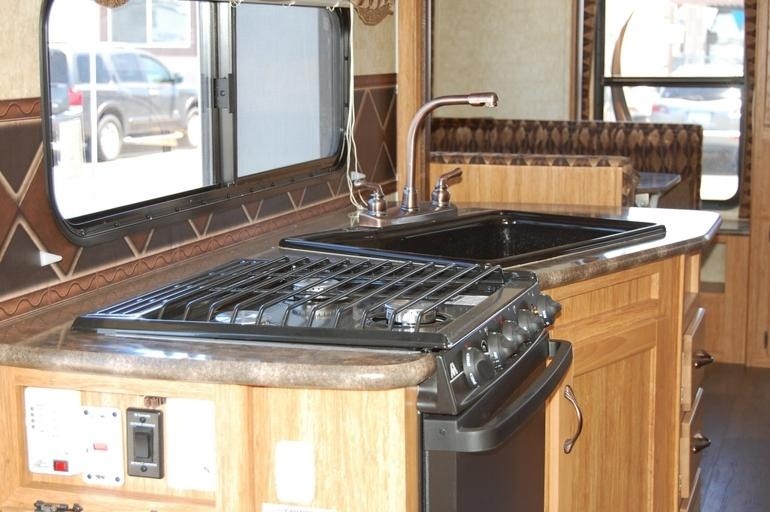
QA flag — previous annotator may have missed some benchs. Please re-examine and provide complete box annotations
[427,152,641,208]
[432,117,704,207]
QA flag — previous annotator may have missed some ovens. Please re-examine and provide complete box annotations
[421,332,575,512]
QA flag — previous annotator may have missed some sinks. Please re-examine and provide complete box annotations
[278,208,667,271]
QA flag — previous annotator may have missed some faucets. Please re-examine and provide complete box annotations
[400,92,498,215]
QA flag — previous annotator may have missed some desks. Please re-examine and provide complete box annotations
[635,172,682,207]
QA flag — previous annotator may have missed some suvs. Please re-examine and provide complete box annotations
[47,39,198,161]
[647,62,742,169]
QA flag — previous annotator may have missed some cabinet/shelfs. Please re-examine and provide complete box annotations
[545,251,713,512]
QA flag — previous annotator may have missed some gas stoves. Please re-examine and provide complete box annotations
[71,256,562,417]
